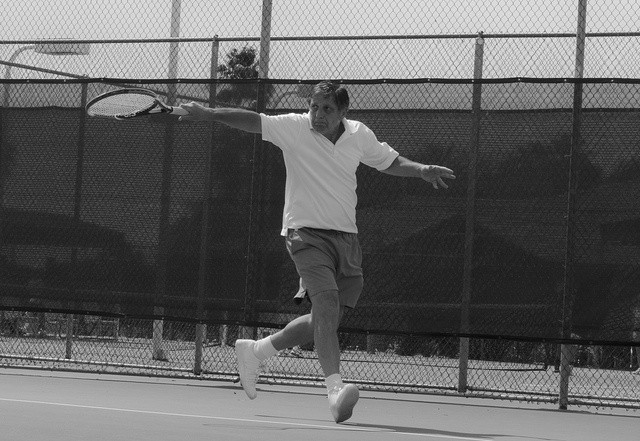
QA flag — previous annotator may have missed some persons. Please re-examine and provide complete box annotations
[176,78,457,425]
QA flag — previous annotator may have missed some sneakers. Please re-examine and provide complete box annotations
[235,338,264,400]
[328,382,360,424]
